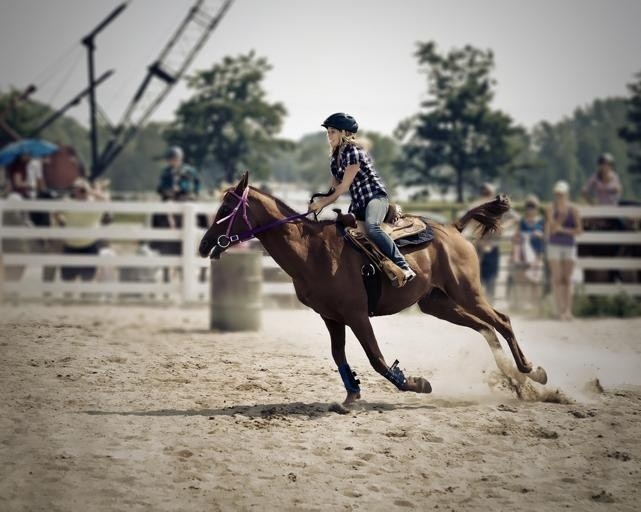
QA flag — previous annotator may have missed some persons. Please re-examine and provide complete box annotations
[545,179,583,323]
[5,147,112,302]
[513,193,549,323]
[308,111,419,287]
[580,150,640,286]
[469,182,521,310]
[117,146,210,296]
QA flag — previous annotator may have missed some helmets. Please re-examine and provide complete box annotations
[166,147,184,160]
[320,113,358,134]
[597,152,613,165]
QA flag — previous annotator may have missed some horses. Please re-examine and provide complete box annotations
[197,169,548,406]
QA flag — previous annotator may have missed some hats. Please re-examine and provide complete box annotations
[553,180,566,193]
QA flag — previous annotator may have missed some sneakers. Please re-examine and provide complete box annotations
[391,267,417,287]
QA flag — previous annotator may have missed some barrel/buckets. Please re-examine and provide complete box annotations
[211,251,263,331]
